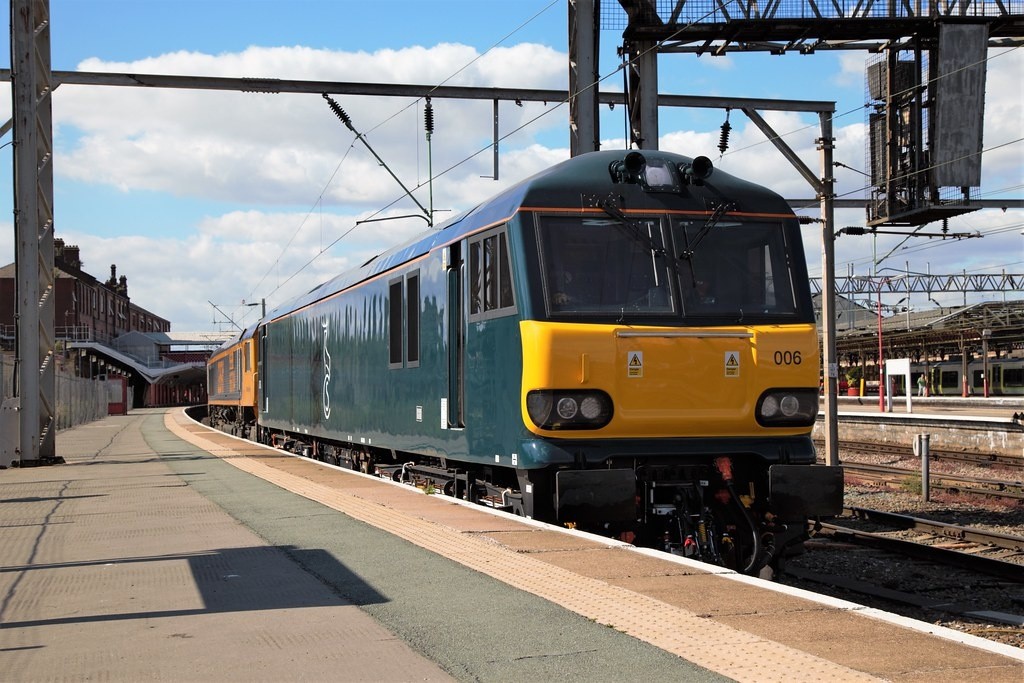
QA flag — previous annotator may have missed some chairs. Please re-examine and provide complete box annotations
[560,255,624,305]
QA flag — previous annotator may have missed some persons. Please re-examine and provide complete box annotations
[917,374,926,397]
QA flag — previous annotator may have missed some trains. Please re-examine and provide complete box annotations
[893,356,1024,397]
[208,149,846,580]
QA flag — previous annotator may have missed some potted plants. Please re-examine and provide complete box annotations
[844,365,866,396]
